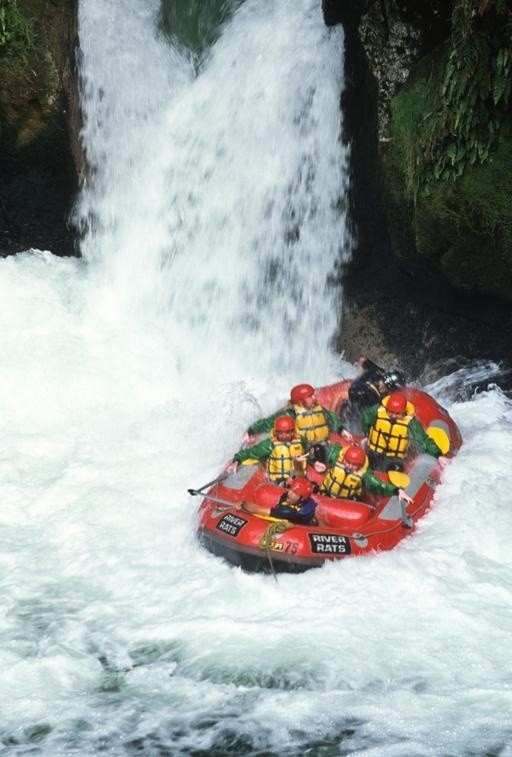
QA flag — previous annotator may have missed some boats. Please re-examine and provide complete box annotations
[197,377,462,577]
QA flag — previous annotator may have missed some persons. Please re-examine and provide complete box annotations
[359,391,451,474]
[295,441,415,504]
[239,383,358,449]
[343,355,405,415]
[232,479,318,526]
[227,414,326,487]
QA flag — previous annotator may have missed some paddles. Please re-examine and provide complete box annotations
[188,458,260,497]
[426,428,450,456]
[380,395,415,418]
[387,469,411,530]
[186,488,291,525]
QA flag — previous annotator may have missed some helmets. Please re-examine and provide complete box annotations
[290,384,314,404]
[274,416,295,431]
[386,393,406,413]
[383,371,404,392]
[345,446,366,465]
[290,478,312,501]
